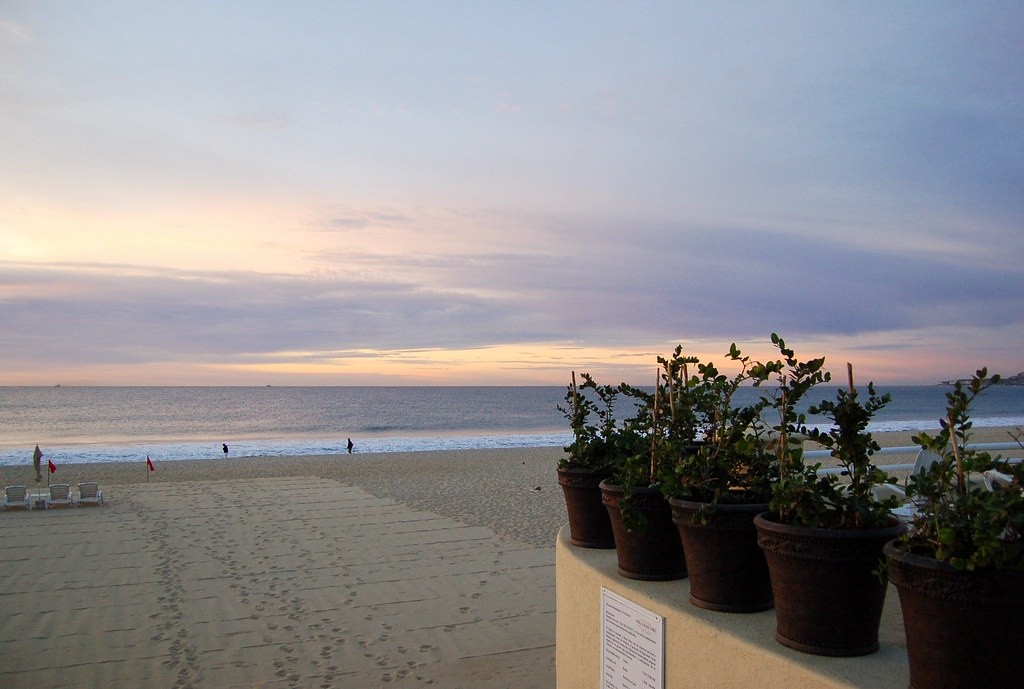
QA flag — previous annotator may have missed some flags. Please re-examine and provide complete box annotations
[147,458,155,471]
[49,461,56,473]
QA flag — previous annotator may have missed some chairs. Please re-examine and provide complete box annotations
[46,484,73,510]
[876,442,953,503]
[76,482,104,508]
[3,486,29,511]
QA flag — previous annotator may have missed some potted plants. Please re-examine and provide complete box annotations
[554,369,647,549]
[650,343,784,613]
[881,367,1024,689]
[752,333,909,658]
[598,345,699,581]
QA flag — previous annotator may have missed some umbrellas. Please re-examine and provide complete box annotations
[33,445,43,481]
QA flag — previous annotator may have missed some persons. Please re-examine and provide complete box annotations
[347,438,353,453]
[223,444,228,459]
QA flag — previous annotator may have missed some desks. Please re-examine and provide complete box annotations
[30,494,48,510]
